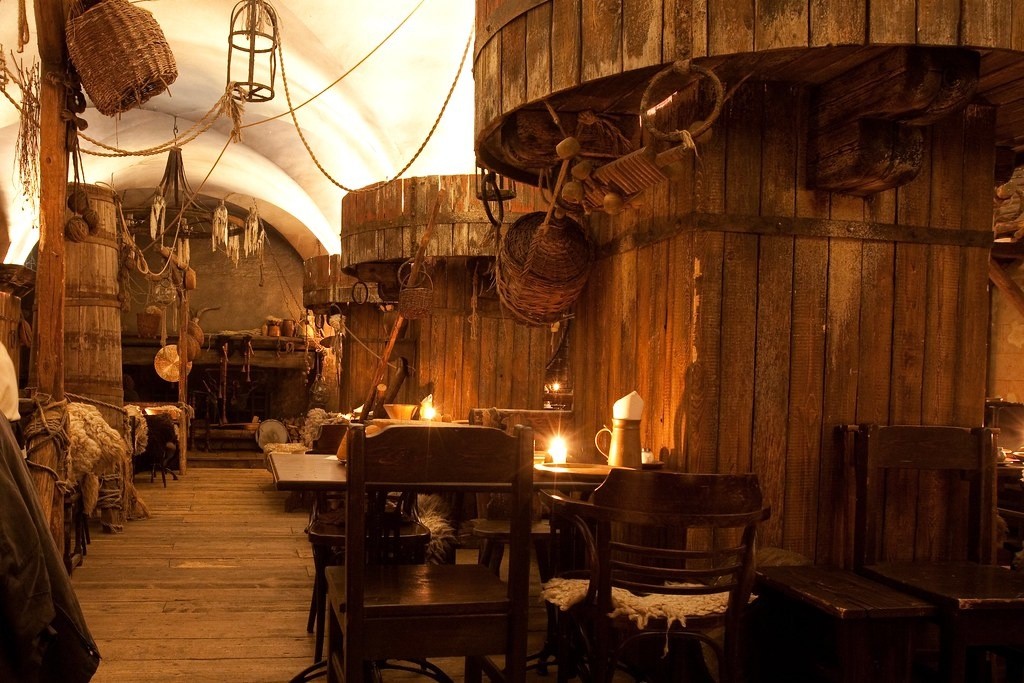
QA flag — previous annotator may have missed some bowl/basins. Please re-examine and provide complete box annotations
[383,404,419,420]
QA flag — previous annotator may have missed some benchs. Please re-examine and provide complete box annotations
[753,564,935,683]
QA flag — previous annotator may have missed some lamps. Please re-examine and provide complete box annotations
[542,353,576,411]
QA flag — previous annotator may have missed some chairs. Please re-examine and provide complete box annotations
[856,422,1024,683]
[324,424,536,683]
[537,466,771,683]
[469,406,576,570]
[307,425,431,664]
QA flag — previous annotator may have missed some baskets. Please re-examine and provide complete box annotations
[65,2,179,115]
[399,272,434,318]
[499,212,596,322]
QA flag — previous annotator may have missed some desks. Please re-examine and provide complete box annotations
[268,452,603,487]
[997,457,1024,547]
[120,333,319,425]
[123,402,190,476]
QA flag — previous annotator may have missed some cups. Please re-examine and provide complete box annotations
[595,419,642,470]
